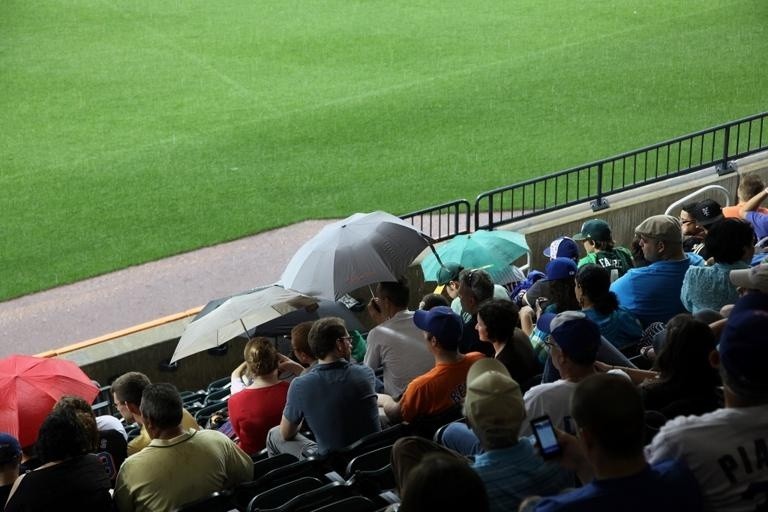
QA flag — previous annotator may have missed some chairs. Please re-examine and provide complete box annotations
[89,343,657,512]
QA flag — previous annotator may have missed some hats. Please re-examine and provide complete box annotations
[464,355,528,434]
[694,198,723,224]
[719,294,767,385]
[633,213,683,242]
[414,305,465,351]
[541,218,613,284]
[536,310,602,366]
[436,262,464,286]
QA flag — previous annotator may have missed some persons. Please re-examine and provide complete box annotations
[1,372,254,511]
[228,175,768,511]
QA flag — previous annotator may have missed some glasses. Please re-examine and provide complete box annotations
[337,334,354,345]
[677,216,692,226]
[543,335,557,349]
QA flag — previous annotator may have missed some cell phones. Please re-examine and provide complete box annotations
[529,415,561,461]
